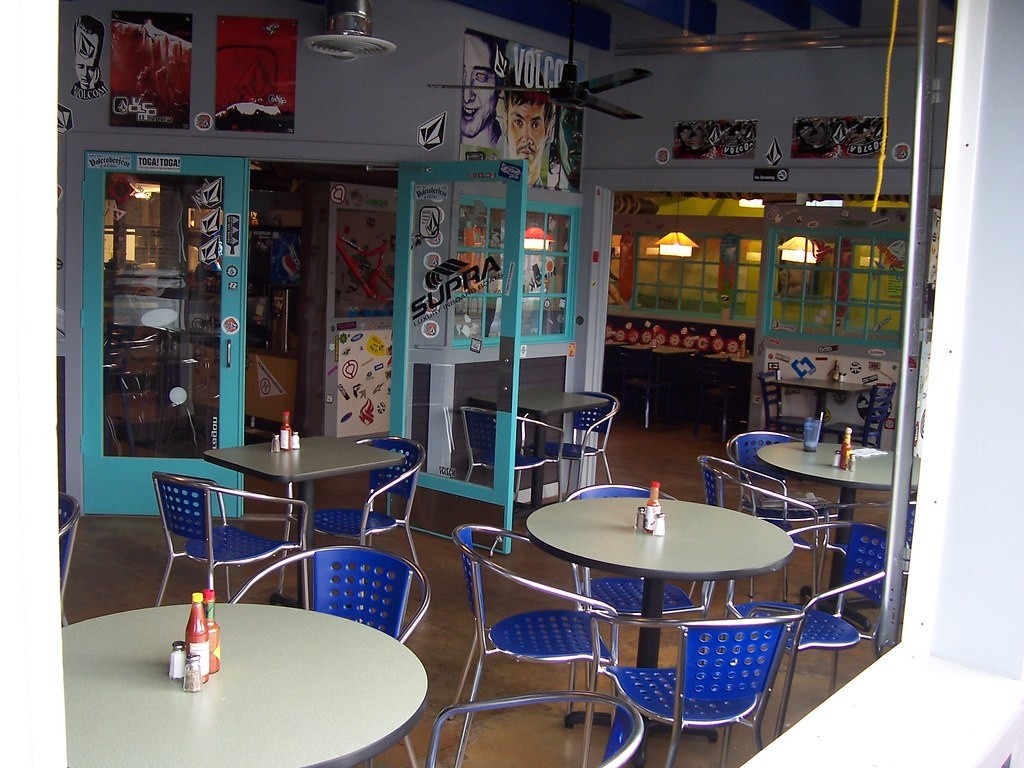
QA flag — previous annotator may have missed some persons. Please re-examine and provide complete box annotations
[462,28,583,193]
[106,242,136,271]
[189,264,217,301]
[248,234,267,296]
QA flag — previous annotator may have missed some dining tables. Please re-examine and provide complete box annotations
[63,604,428,768]
[527,499,795,768]
[200,436,405,610]
[467,389,609,519]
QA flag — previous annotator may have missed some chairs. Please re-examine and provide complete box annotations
[60,372,920,768]
[619,351,739,444]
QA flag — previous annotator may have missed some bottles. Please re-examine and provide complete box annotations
[291,432,300,449]
[833,427,856,472]
[832,361,845,383]
[653,513,666,537]
[183,654,202,692]
[202,589,220,674]
[185,593,209,684]
[271,434,280,453]
[635,507,644,531]
[169,640,186,680]
[644,481,662,533]
[776,369,783,381]
[280,411,292,451]
[606,332,746,358]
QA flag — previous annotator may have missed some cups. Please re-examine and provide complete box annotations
[803,417,822,452]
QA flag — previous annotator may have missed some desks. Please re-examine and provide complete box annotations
[756,443,921,632]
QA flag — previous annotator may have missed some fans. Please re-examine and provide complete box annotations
[426,0,653,121]
